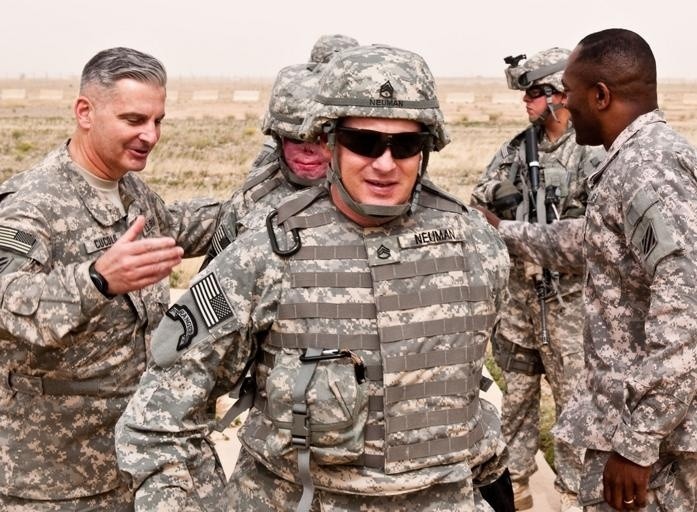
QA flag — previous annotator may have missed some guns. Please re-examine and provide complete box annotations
[487,127,560,346]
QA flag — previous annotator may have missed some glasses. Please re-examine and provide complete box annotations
[336,125,426,160]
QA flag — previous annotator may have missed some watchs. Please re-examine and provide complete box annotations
[89,264,117,299]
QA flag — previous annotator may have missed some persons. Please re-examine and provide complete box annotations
[113,44,516,512]
[476,27,697,512]
[0,47,225,512]
[253,34,359,167]
[470,46,603,512]
[166,61,326,258]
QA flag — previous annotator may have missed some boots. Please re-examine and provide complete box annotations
[510,479,532,509]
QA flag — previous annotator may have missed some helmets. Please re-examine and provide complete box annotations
[306,35,359,61]
[297,42,450,152]
[262,61,324,142]
[526,46,572,95]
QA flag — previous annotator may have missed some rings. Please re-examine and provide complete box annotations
[623,496,637,504]
